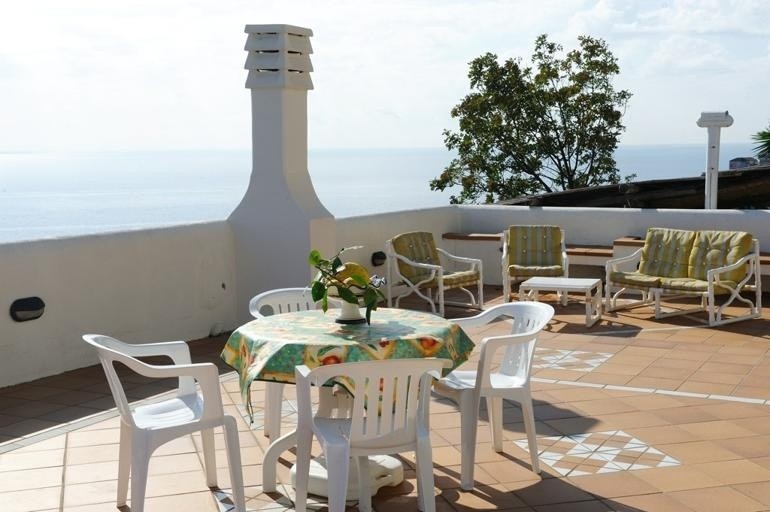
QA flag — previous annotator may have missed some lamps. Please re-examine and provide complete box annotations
[10,296,46,322]
[372,251,386,267]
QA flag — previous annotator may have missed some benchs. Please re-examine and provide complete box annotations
[566,246,613,267]
[614,235,645,272]
[443,230,505,286]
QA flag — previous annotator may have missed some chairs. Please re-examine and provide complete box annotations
[605,229,762,328]
[412,301,556,491]
[385,230,484,318]
[248,287,347,447]
[82,333,246,511]
[502,224,569,307]
[263,357,453,512]
